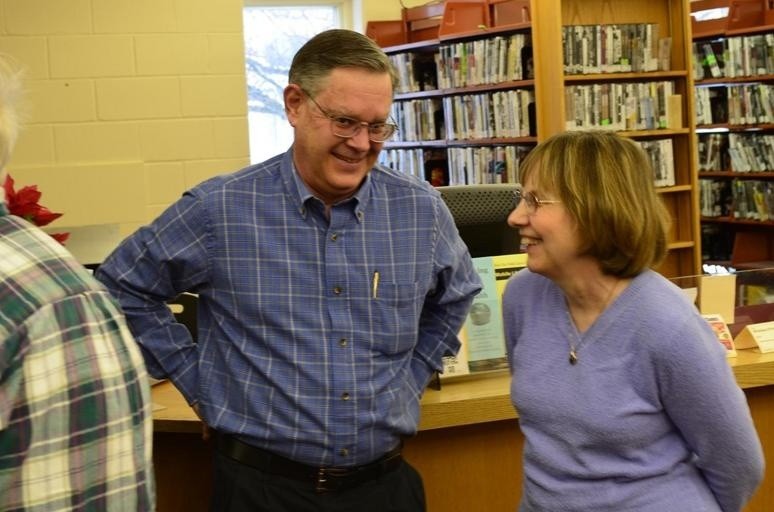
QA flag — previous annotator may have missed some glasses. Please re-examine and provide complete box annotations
[300,89,399,143]
[511,191,565,211]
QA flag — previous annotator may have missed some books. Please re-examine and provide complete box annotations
[561,23,683,189]
[692,34,774,260]
[376,33,534,187]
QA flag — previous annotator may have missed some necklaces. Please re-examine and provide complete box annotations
[559,276,622,365]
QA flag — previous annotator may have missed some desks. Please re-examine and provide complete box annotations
[34,220,124,277]
[145,334,774,511]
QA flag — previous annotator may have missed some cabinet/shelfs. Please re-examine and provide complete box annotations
[364,0,774,308]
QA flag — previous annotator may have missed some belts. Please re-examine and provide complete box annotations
[201,421,403,494]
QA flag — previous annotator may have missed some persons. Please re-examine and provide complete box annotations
[0,52,157,512]
[95,29,484,512]
[501,129,766,512]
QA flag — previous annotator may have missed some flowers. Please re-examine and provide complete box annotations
[0,172,71,251]
